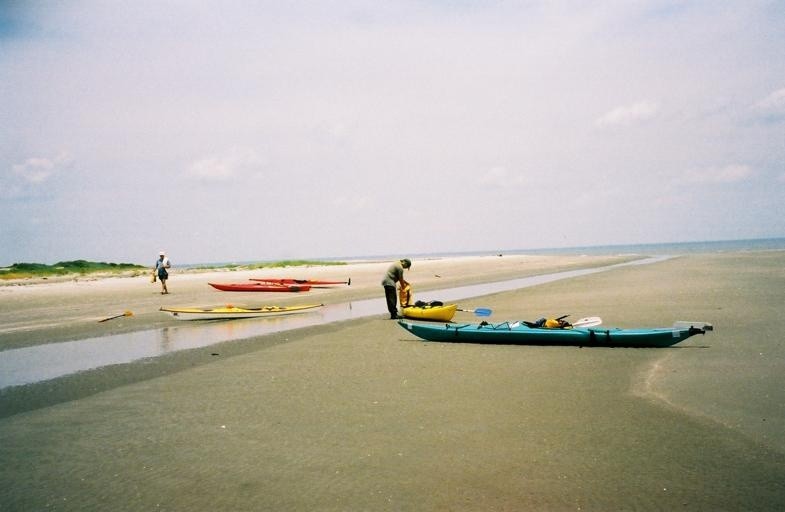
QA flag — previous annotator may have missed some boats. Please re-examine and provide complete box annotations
[161,300,329,323]
[249,277,353,290]
[397,298,459,321]
[394,316,708,351]
[205,280,311,293]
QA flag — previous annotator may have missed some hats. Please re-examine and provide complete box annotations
[160,252,164,255]
[404,258,411,270]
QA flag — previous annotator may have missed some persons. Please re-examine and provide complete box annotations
[149,253,171,295]
[382,258,411,319]
